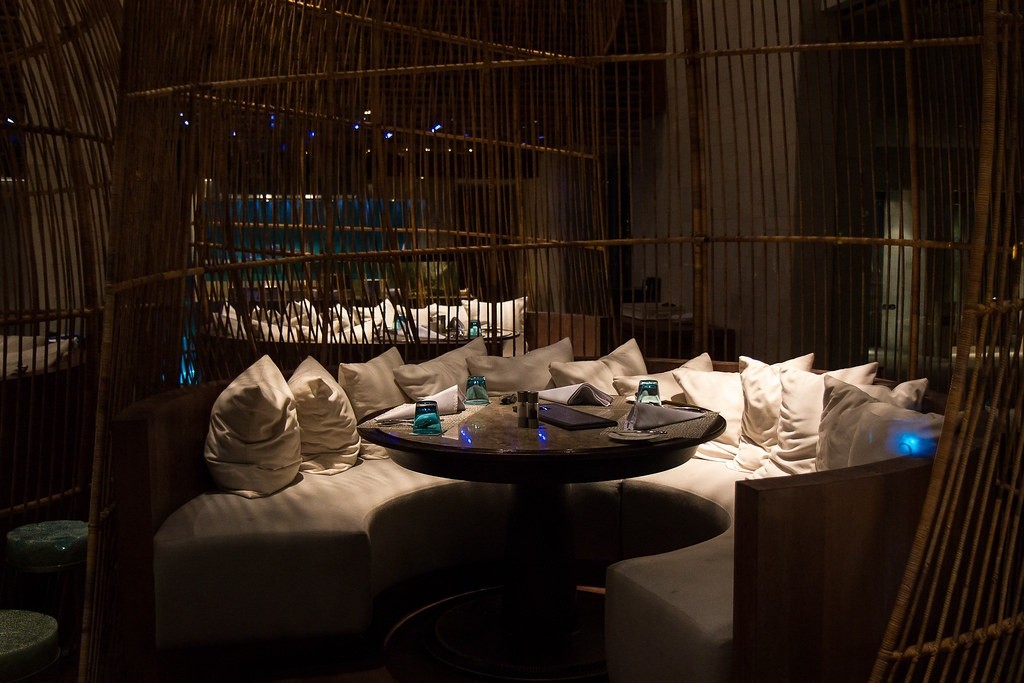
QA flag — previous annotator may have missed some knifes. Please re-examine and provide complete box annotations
[605,430,668,434]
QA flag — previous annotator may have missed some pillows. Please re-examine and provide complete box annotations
[194,335,979,500]
[207,297,529,334]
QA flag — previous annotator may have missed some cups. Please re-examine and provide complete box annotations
[413,401,442,434]
[470,321,482,337]
[636,380,662,407]
[464,376,490,405]
[396,316,403,329]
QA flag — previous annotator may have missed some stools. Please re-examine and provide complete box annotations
[0,607,61,683]
[7,520,90,660]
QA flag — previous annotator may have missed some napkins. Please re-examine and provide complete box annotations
[536,382,612,407]
[374,384,466,425]
[629,402,704,429]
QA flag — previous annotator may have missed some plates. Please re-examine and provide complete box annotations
[626,396,666,403]
[607,429,659,442]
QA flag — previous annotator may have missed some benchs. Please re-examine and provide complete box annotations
[200,310,531,358]
[124,357,1024,683]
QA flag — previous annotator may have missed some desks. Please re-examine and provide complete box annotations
[357,396,726,683]
[373,327,526,345]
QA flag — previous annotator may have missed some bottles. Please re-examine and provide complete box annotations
[517,390,540,429]
